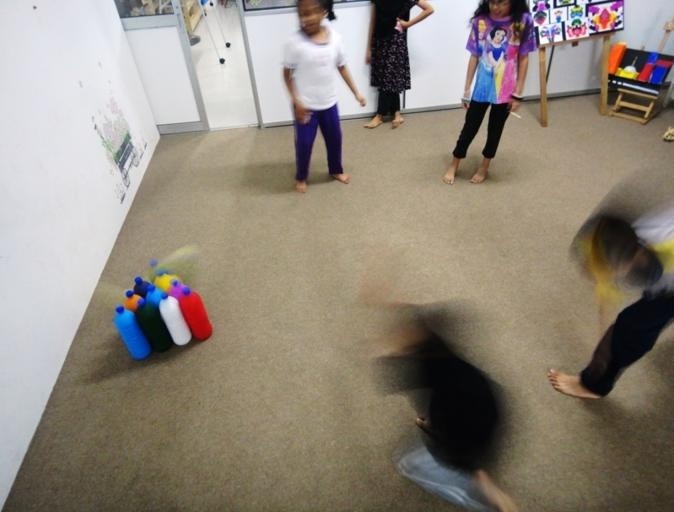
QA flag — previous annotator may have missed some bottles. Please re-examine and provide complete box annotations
[111,259,211,359]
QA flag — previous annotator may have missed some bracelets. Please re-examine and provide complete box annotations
[510,91,525,102]
[461,94,471,102]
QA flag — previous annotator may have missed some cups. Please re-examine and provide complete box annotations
[608,43,672,84]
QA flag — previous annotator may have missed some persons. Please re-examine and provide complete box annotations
[442,1,537,184]
[282,0,367,193]
[368,305,522,512]
[365,1,434,129]
[547,200,674,399]
